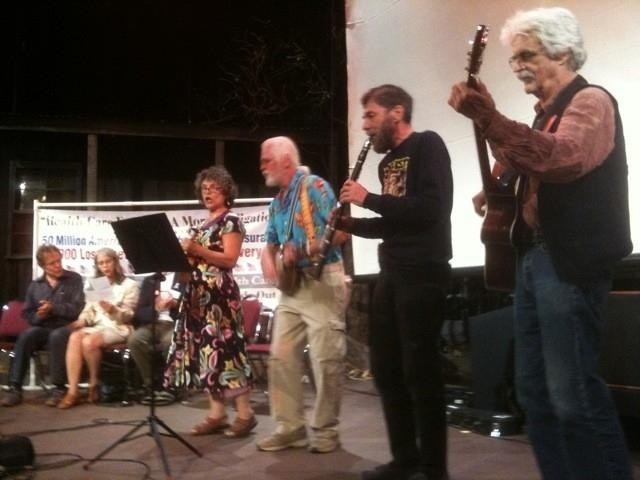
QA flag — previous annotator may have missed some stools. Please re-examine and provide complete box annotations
[246,344,271,391]
[101,341,134,407]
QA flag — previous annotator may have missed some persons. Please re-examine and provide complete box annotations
[448,5,638,479]
[126,269,191,406]
[57,248,140,410]
[0,244,87,406]
[257,135,350,453]
[162,166,258,437]
[324,84,453,479]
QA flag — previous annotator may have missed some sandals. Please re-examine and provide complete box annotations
[191,416,230,435]
[226,416,257,440]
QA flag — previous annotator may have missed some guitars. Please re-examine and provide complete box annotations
[172,224,199,297]
[464,25,523,295]
[275,239,323,296]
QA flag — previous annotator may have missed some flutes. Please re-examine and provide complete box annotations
[313,134,373,282]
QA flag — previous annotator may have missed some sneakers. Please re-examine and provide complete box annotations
[45,388,99,408]
[363,462,447,479]
[257,424,308,452]
[2,389,24,405]
[309,436,340,453]
[142,391,174,405]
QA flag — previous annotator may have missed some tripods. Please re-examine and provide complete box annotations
[83,265,202,480]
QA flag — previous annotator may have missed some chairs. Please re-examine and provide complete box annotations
[240,295,274,389]
[1,298,50,394]
